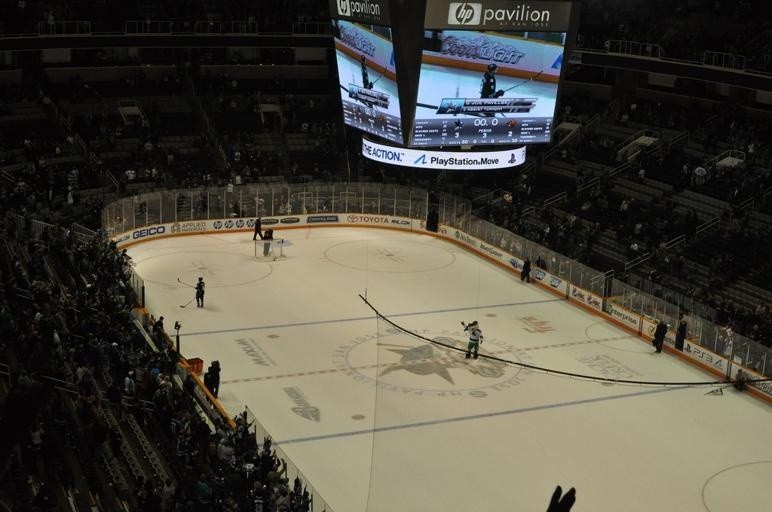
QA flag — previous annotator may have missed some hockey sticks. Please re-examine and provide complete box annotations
[177,277,195,288]
[179,298,197,308]
[504,71,542,93]
[371,67,386,85]
